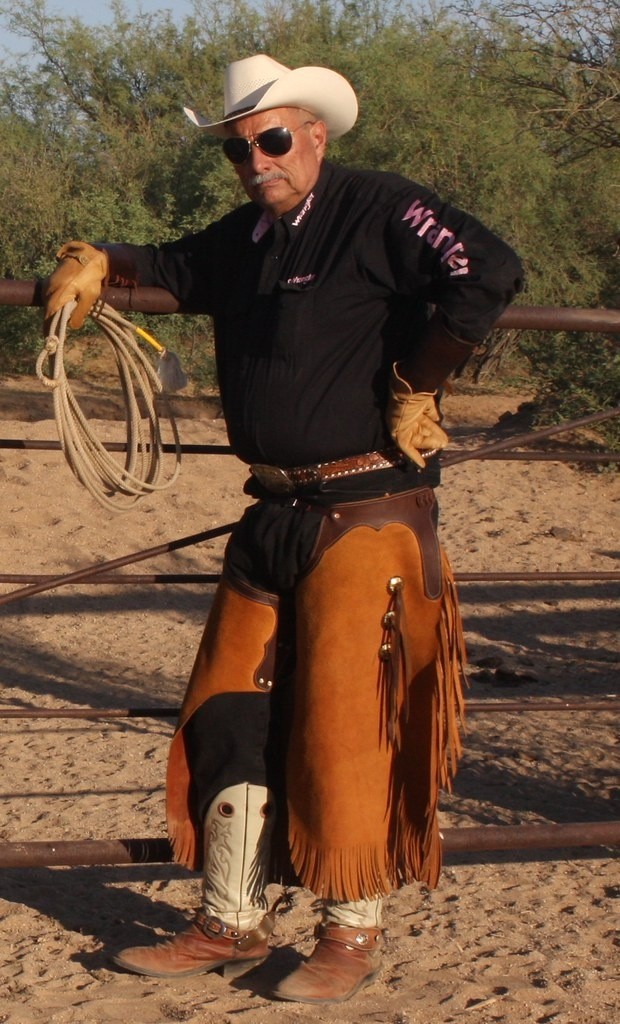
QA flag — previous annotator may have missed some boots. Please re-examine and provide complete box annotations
[272,886,384,1006]
[112,782,277,979]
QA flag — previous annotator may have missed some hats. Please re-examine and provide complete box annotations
[183,54,358,144]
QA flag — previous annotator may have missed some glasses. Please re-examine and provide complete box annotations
[222,121,317,165]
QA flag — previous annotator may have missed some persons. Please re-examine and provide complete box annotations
[36,52,529,1001]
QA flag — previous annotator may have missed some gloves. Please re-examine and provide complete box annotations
[387,361,449,469]
[42,241,107,340]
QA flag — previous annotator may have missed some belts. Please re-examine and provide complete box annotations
[248,448,440,497]
